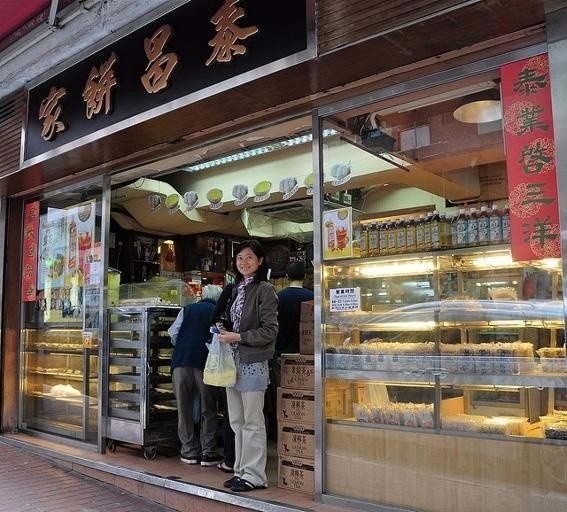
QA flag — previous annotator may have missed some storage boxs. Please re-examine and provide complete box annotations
[274,299,314,494]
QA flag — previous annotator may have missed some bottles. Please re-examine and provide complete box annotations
[359,203,509,257]
[327,223,336,248]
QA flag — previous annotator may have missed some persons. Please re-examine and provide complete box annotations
[267,257,313,442]
[212,283,240,472]
[168,284,224,466]
[214,239,279,491]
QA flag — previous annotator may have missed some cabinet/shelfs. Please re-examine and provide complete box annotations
[15,304,188,462]
[320,241,565,445]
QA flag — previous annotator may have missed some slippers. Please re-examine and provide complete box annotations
[217,462,235,473]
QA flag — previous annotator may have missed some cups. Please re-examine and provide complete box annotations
[335,227,349,249]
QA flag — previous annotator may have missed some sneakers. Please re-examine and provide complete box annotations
[224,476,241,488]
[180,455,198,464]
[231,479,268,492]
[201,454,224,467]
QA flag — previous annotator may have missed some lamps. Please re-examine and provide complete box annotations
[449,88,506,125]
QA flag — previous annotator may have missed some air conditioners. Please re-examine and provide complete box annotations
[249,186,366,224]
